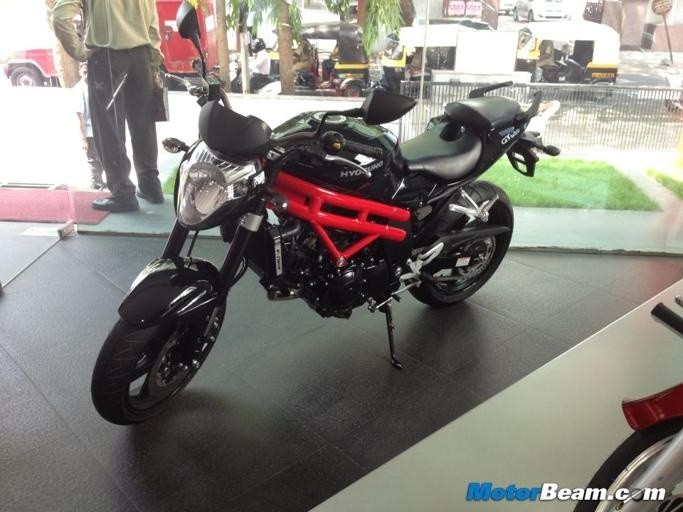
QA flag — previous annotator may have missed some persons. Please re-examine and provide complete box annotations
[51,1,165,215]
[69,60,107,190]
[247,38,270,93]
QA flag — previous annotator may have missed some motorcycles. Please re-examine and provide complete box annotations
[231,20,624,101]
[92,0,562,431]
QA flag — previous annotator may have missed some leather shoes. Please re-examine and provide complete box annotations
[137,187,164,203]
[92,196,140,211]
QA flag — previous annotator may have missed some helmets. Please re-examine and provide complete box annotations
[252,38,264,53]
